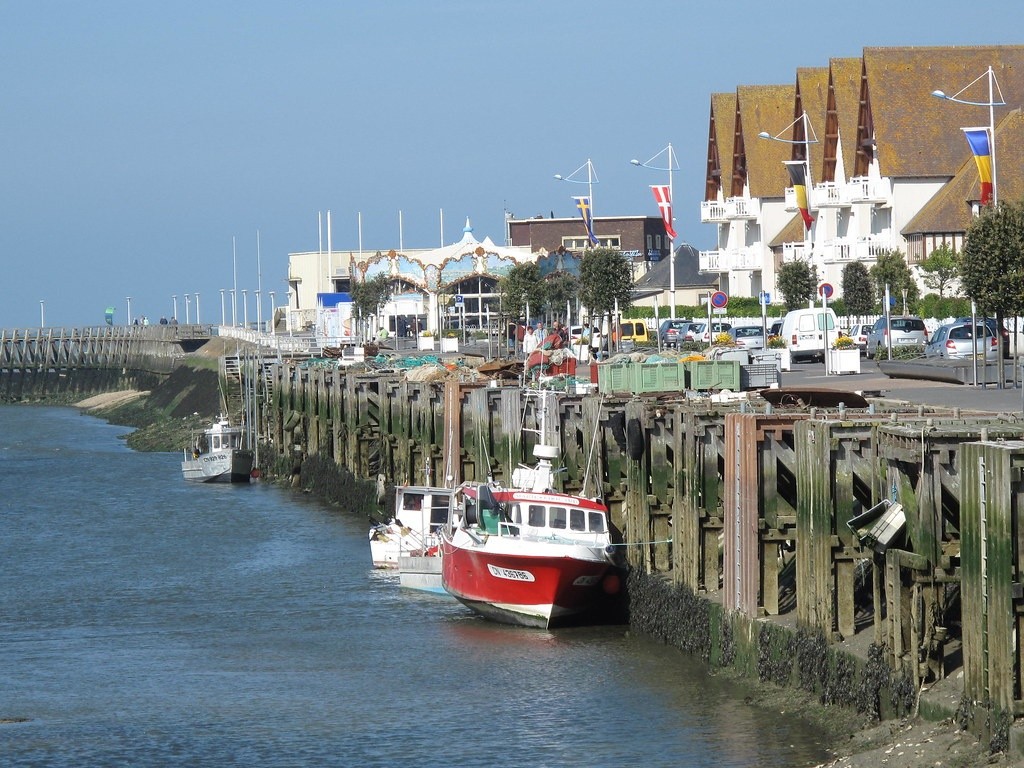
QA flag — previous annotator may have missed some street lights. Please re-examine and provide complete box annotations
[629,160,674,318]
[194,293,200,325]
[40,300,45,328]
[219,289,225,326]
[269,291,276,336]
[930,90,1008,213]
[553,175,599,253]
[229,290,235,326]
[285,292,293,337]
[758,132,820,268]
[126,297,131,326]
[241,290,248,328]
[254,290,261,332]
[172,295,177,320]
[184,294,189,324]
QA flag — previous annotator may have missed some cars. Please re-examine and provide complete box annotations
[656,320,694,349]
[676,324,706,352]
[571,326,606,361]
[692,323,732,343]
[953,317,1010,360]
[866,316,929,360]
[770,319,784,337]
[847,324,874,350]
[923,322,997,366]
[728,326,772,356]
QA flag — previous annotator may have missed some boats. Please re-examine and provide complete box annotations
[398,533,448,594]
[367,458,462,568]
[181,412,255,484]
[440,385,626,630]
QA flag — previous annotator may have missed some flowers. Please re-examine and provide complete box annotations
[716,331,734,345]
[576,337,589,345]
[448,332,455,338]
[768,334,789,349]
[832,334,855,349]
[423,330,431,337]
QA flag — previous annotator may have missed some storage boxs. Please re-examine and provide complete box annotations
[690,359,742,392]
[590,361,598,383]
[597,363,636,392]
[739,363,780,388]
[750,353,781,371]
[635,362,686,394]
[550,358,576,377]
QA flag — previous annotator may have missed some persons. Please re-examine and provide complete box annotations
[169,317,178,324]
[143,317,149,325]
[551,322,565,343]
[160,316,168,324]
[133,318,138,324]
[521,327,540,356]
[535,322,549,342]
[140,316,144,325]
[583,324,605,365]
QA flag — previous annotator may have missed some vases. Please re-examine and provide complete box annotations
[419,336,434,351]
[828,347,862,376]
[574,344,589,362]
[442,337,459,353]
[717,346,749,364]
[766,348,791,371]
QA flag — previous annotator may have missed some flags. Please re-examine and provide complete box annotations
[785,164,815,230]
[574,198,600,247]
[965,130,992,203]
[652,187,677,241]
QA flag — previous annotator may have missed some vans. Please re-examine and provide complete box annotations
[778,308,841,364]
[620,319,649,342]
